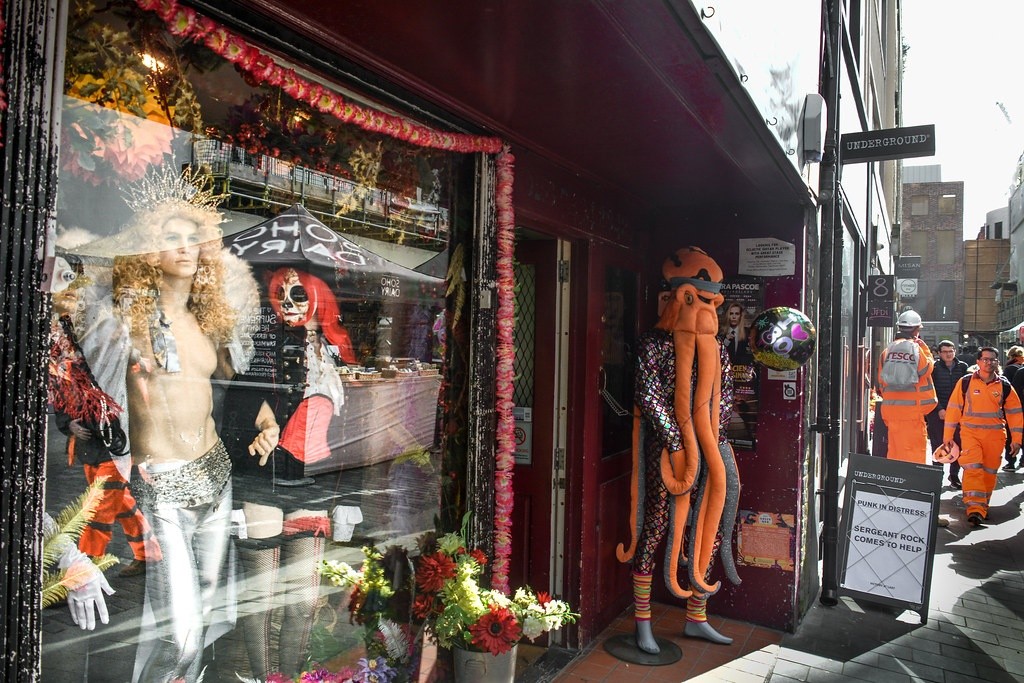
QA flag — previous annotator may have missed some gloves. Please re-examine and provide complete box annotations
[44,514,115,631]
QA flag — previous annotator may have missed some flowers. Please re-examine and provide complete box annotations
[264,656,397,683]
[413,511,581,656]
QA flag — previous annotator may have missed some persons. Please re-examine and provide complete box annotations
[630,248,740,655]
[926,340,969,489]
[720,302,751,366]
[220,267,355,682]
[51,319,162,631]
[877,309,950,528]
[944,347,1024,526]
[50,246,115,630]
[928,343,984,366]
[1002,344,1023,471]
[51,196,255,683]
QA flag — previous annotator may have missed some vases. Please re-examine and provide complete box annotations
[453,647,517,683]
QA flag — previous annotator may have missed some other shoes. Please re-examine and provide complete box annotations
[119,559,159,578]
[44,586,76,603]
[938,518,949,526]
[968,513,982,525]
[1003,465,1015,471]
[985,514,990,520]
[1019,463,1024,466]
[948,476,962,489]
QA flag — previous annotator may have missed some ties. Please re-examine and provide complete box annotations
[729,331,735,354]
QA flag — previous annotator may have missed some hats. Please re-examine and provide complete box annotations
[1007,346,1024,356]
[283,251,338,290]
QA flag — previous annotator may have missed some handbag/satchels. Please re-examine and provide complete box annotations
[881,341,919,387]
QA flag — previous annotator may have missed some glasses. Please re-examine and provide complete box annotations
[980,357,997,363]
[942,349,956,353]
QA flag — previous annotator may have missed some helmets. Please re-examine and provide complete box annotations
[932,441,960,464]
[896,310,923,329]
